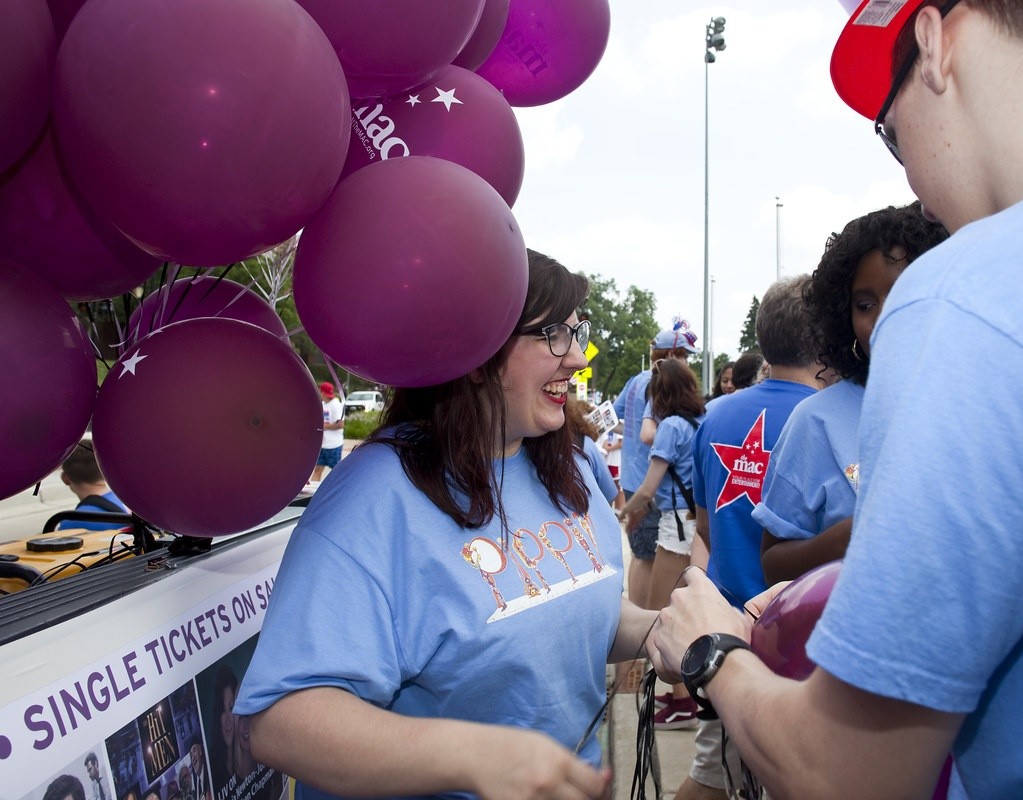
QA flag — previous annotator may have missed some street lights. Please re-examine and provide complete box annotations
[703,16,728,398]
[776,196,782,283]
[709,275,715,398]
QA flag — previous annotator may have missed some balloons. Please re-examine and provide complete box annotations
[0,0,611,539]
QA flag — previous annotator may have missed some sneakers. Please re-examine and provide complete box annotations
[653,706,700,729]
[655,692,673,708]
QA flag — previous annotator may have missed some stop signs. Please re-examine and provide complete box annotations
[580,385,585,390]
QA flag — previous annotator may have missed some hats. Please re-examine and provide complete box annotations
[651,330,699,353]
[830,0,942,118]
[320,383,336,398]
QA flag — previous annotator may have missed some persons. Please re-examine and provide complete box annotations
[562,196,951,800]
[83,752,113,800]
[58,440,132,533]
[204,664,240,794]
[652,0,1023,800]
[313,380,345,483]
[232,684,282,800]
[118,742,212,800]
[232,250,666,799]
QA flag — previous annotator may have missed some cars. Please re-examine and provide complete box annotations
[344,391,386,417]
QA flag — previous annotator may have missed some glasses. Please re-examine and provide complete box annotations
[514,320,591,356]
[875,0,957,165]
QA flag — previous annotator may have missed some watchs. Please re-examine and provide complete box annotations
[680,633,755,722]
